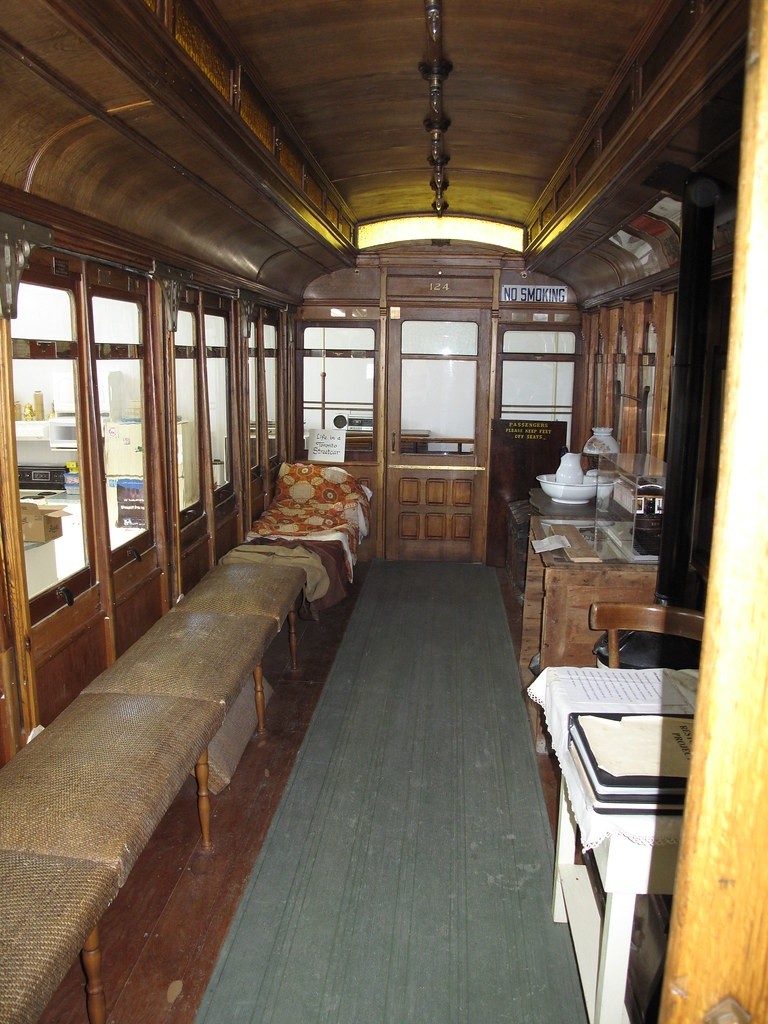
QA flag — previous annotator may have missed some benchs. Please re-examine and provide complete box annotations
[0,501,371,1024]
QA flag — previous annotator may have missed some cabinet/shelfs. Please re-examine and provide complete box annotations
[344,429,432,452]
[520,516,659,754]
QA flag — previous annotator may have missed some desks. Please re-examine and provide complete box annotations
[525,666,707,1023]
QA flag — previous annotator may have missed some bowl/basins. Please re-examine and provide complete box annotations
[537,474,597,505]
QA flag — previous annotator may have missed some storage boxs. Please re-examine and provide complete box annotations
[115,479,148,529]
[19,503,73,543]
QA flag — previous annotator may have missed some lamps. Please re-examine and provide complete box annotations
[415,0,453,219]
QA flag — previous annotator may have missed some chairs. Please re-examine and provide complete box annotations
[588,602,704,671]
